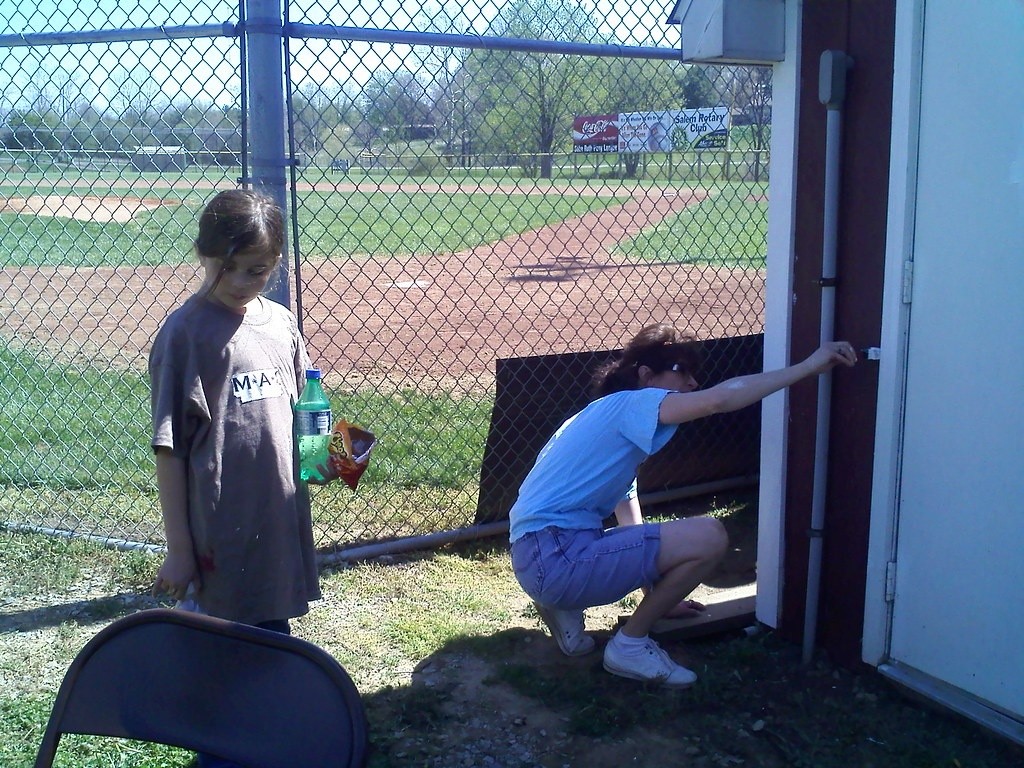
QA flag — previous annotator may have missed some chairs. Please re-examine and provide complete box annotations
[34,607,368,768]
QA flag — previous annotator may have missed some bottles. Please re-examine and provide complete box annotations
[173,582,208,616]
[294,370,333,480]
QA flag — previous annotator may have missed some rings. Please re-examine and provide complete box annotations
[689,600,694,604]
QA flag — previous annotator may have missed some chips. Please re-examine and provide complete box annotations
[350,438,364,457]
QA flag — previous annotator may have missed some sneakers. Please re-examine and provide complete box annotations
[603,636,697,689]
[534,601,595,657]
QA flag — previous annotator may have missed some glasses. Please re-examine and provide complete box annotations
[651,359,699,377]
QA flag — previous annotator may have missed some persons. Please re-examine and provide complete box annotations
[510,326,858,690]
[148,191,340,636]
